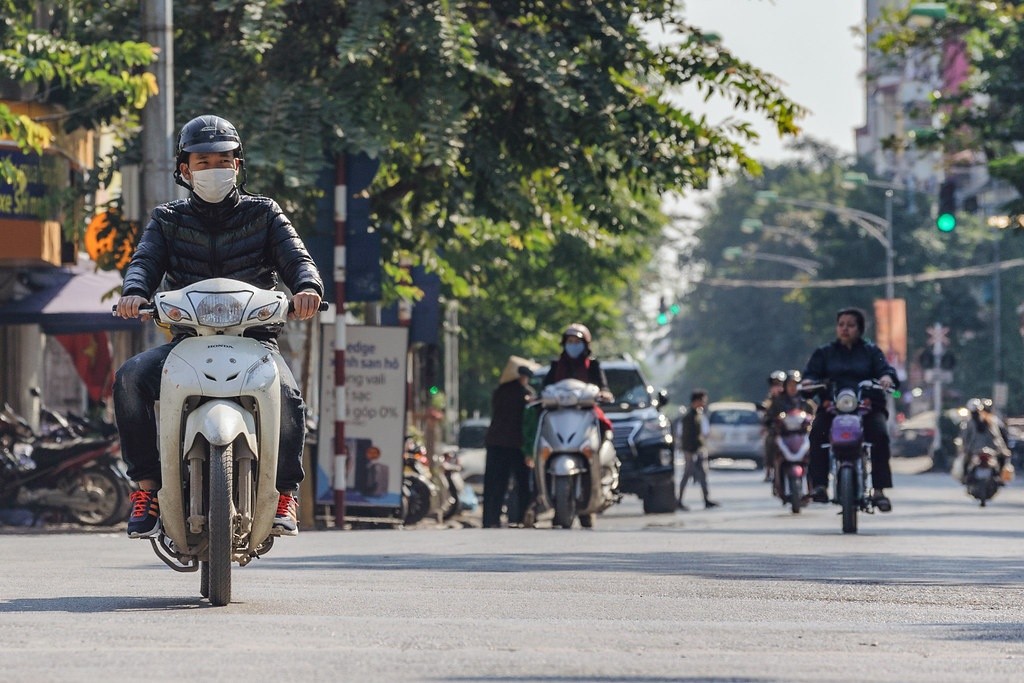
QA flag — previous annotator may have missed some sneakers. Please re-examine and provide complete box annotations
[127,485,161,538]
[271,493,300,536]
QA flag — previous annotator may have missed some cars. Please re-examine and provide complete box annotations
[899,409,946,458]
[456,419,493,493]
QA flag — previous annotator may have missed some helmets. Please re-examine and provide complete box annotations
[559,321,592,343]
[176,115,244,167]
[768,369,802,383]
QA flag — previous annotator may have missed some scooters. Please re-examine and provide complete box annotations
[524,377,621,528]
[953,436,1005,507]
[756,401,818,512]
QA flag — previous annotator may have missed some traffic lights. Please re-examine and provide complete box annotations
[422,344,438,402]
[658,304,667,325]
[937,181,956,233]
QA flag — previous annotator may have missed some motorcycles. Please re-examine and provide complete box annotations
[796,377,895,532]
[0,385,139,529]
[348,434,460,526]
[114,275,330,605]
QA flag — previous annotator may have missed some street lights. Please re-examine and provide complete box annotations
[752,190,895,453]
[840,170,1001,411]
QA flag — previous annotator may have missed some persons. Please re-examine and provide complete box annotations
[802,306,901,512]
[110,115,324,535]
[482,365,536,528]
[981,398,1008,468]
[528,323,614,454]
[677,389,719,510]
[761,370,817,485]
[962,398,1011,482]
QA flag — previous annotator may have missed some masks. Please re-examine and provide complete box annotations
[564,341,586,358]
[185,162,237,204]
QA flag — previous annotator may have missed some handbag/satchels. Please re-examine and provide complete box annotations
[999,457,1015,482]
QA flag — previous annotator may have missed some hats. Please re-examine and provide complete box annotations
[968,397,992,412]
[499,353,543,385]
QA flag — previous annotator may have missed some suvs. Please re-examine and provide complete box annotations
[701,403,767,468]
[596,352,676,514]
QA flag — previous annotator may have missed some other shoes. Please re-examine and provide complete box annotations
[814,487,829,504]
[874,495,892,511]
